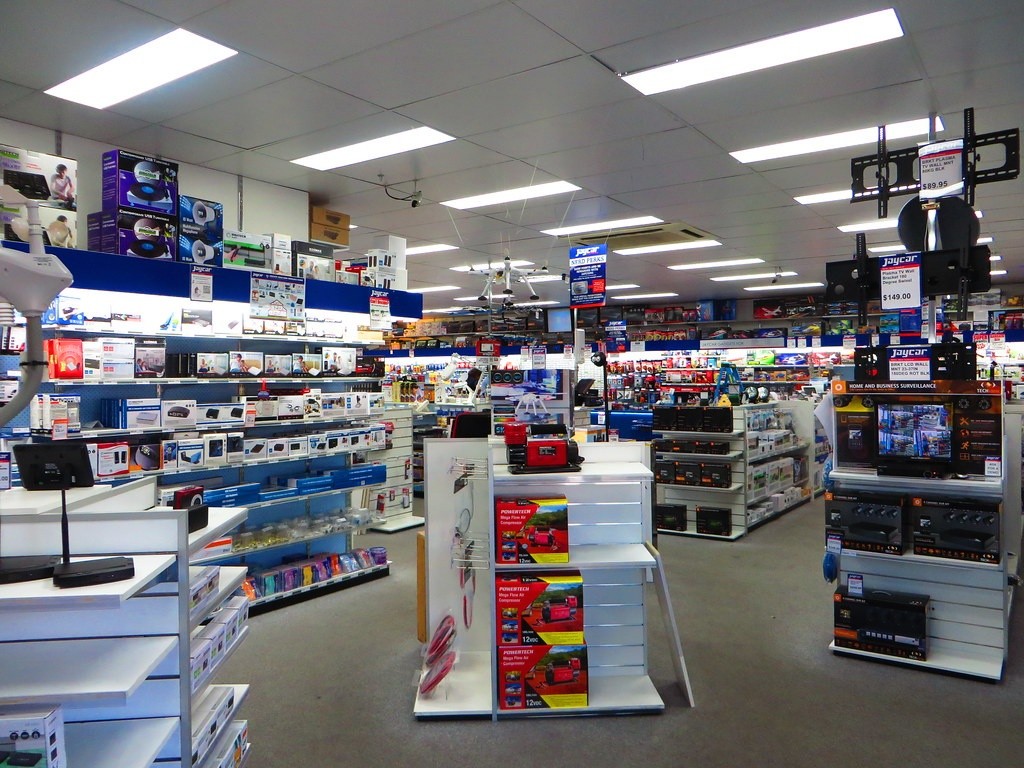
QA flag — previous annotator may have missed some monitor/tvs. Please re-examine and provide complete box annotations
[873,402,955,477]
[544,306,574,333]
[14,441,95,492]
[575,379,594,394]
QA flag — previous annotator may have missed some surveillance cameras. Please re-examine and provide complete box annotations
[412,191,422,208]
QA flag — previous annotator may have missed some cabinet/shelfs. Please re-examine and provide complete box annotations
[0,509,250,768]
[661,362,858,405]
[648,401,814,541]
[413,437,670,720]
[3,329,395,612]
[821,372,1024,681]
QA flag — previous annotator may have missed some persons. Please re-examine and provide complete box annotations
[293,356,308,373]
[50,164,75,201]
[199,359,208,372]
[330,352,338,365]
[230,354,248,372]
[136,358,147,372]
[266,360,274,372]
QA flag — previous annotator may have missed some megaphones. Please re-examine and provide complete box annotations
[191,240,220,264]
[192,201,220,232]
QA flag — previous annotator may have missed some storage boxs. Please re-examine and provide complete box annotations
[822,488,1002,662]
[585,411,620,442]
[188,564,247,768]
[649,408,805,536]
[0,702,69,768]
[491,497,590,710]
[0,148,402,506]
